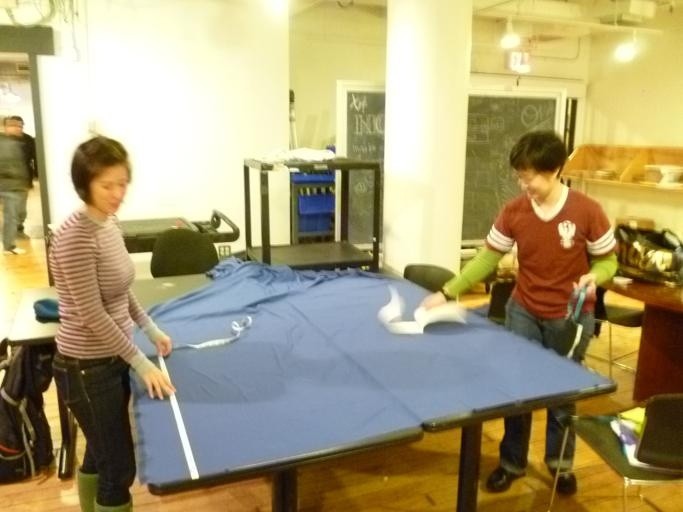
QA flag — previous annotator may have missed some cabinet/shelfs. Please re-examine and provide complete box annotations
[243,156,385,273]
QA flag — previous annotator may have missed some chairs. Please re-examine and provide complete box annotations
[403,264,457,303]
[543,391,682,511]
[149,229,219,277]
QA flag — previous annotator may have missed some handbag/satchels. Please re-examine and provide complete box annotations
[614,223,683,288]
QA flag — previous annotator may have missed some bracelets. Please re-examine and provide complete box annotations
[440,289,450,301]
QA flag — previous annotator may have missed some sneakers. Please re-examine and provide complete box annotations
[3,247,27,256]
[16,230,30,240]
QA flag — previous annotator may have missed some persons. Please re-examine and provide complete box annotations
[48,133,177,512]
[419,129,620,496]
[0,115,37,257]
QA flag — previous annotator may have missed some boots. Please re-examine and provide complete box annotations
[78,466,133,512]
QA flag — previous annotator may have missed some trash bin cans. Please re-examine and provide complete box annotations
[487,273,517,318]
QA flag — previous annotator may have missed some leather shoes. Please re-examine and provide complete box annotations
[547,466,578,498]
[486,465,527,493]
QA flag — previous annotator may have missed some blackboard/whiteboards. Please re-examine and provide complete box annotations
[333,82,569,248]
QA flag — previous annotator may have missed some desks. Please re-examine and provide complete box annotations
[128,254,424,512]
[5,281,78,480]
[603,277,682,403]
[235,248,617,512]
